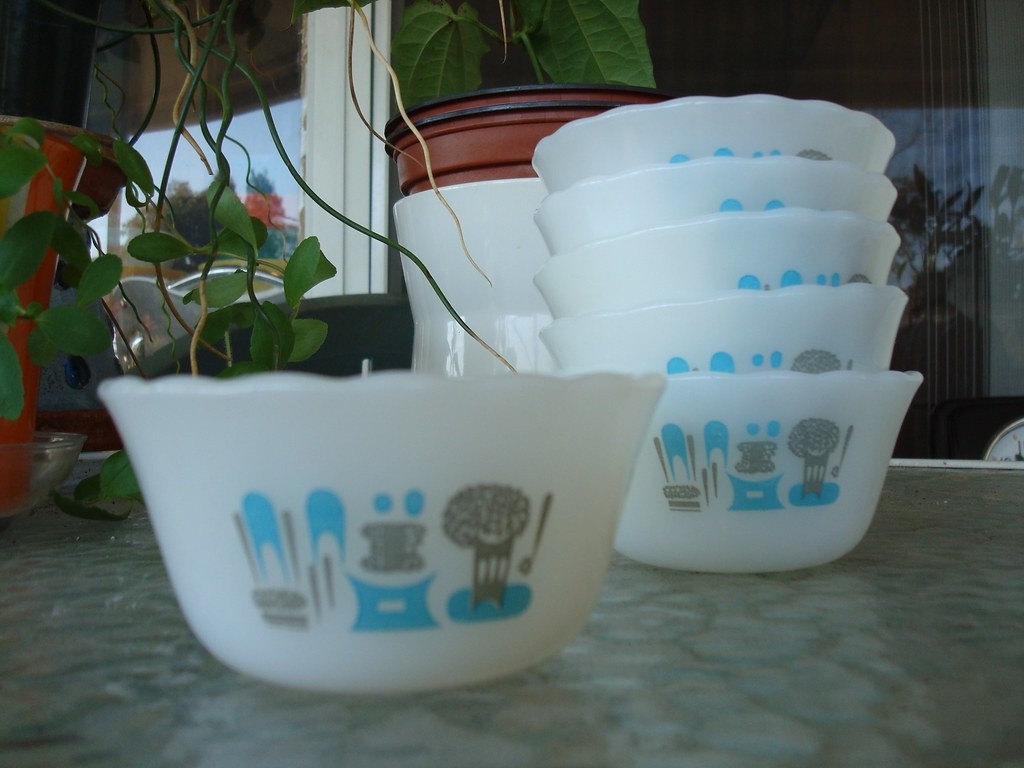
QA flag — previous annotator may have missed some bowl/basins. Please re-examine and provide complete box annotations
[1,430,89,516]
[531,94,924,580]
[97,371,670,699]
[392,174,551,374]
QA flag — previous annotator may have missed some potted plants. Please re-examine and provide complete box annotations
[0,0,529,523]
[286,0,671,195]
